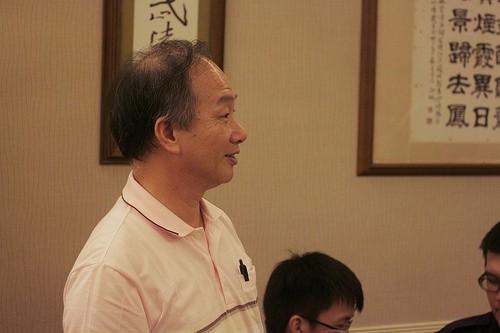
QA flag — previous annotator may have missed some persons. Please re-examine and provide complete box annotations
[435,222,500,333]
[263,250,364,333]
[62,39,264,333]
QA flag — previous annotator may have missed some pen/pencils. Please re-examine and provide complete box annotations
[238,259,250,281]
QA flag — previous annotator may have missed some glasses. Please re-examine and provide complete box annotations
[304,316,353,331]
[478,272,500,292]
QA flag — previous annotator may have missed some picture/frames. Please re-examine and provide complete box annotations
[100,0,224,165]
[356,0,500,176]
